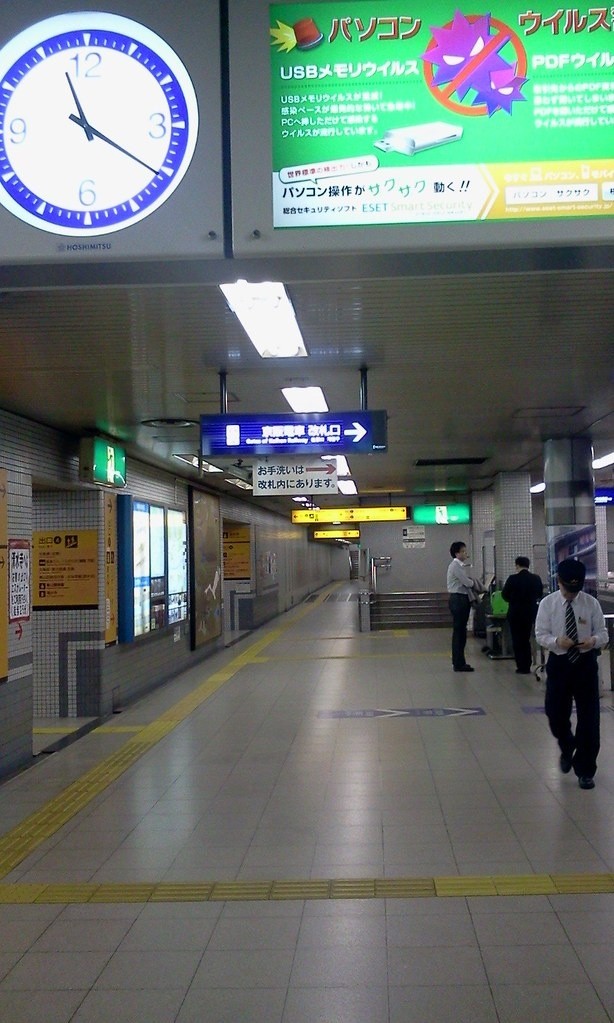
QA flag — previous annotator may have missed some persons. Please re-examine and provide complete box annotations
[447,542,475,672]
[535,558,609,789]
[501,557,544,674]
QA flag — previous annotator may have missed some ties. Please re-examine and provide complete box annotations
[564,599,580,663]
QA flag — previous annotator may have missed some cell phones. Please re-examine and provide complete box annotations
[573,643,585,647]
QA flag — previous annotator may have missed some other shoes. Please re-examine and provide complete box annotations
[560,744,573,773]
[453,663,474,673]
[577,773,595,789]
[516,667,532,673]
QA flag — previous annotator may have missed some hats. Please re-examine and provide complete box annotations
[559,559,586,592]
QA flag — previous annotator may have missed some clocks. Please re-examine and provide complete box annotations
[0,11,199,235]
